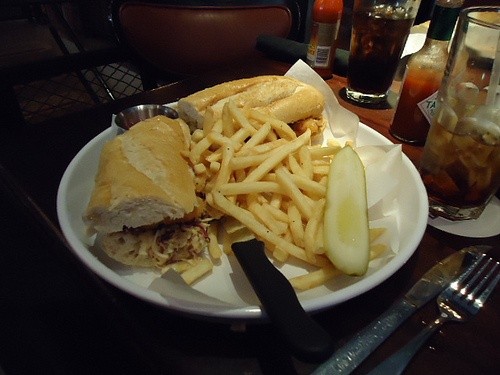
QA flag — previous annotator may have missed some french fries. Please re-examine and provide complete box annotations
[161,101,387,290]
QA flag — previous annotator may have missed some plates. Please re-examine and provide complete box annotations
[428,194,500,238]
[57,102,429,318]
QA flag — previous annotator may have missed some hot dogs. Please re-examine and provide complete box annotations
[84,116,208,267]
[179,74,326,135]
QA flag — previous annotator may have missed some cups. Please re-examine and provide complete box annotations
[346,0,422,105]
[418,5,500,221]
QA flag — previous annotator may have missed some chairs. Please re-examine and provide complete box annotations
[108,0,314,92]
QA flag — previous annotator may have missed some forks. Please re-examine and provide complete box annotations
[368,253,500,375]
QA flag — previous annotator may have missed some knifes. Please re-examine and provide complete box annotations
[178,100,333,364]
[313,244,494,375]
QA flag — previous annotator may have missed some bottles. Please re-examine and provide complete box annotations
[306,0,344,80]
[389,1,465,147]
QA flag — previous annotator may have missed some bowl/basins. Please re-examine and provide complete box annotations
[115,104,178,136]
